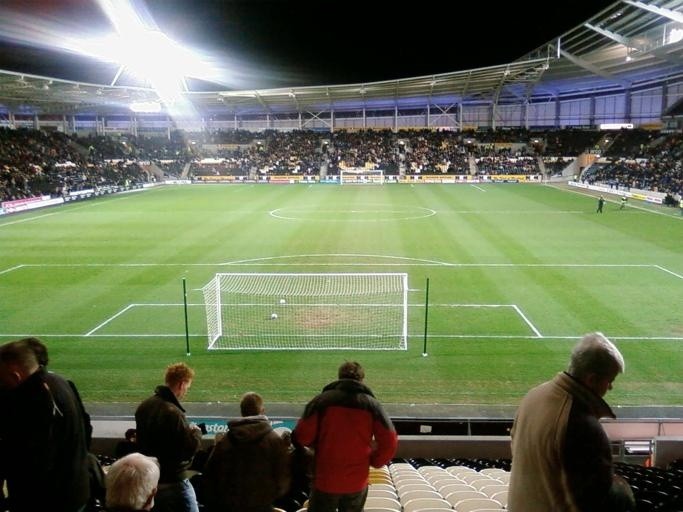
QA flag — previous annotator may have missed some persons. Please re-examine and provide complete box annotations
[0,337,400,511]
[595,195,605,215]
[0,125,683,209]
[619,193,627,211]
[506,331,635,512]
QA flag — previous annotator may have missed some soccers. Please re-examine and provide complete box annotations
[280,298,285,305]
[271,313,277,319]
